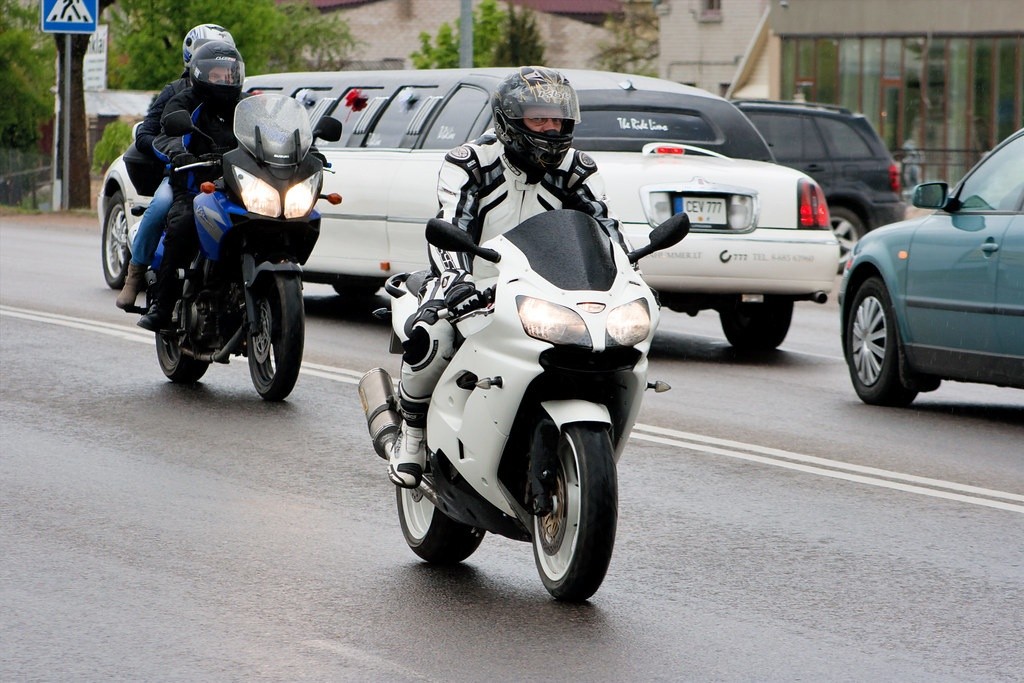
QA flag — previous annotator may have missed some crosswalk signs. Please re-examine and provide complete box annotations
[42,1,98,34]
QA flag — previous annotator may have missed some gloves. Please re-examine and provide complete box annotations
[440,269,484,318]
[312,153,327,167]
[649,287,662,310]
[172,153,195,170]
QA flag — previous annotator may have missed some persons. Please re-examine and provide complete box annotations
[115,24,327,307]
[387,66,643,488]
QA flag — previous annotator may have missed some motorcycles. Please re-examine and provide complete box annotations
[125,97,342,402]
[357,213,693,603]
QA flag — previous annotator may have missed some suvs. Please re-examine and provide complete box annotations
[730,99,907,275]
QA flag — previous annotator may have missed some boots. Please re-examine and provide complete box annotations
[387,381,432,489]
[116,263,147,308]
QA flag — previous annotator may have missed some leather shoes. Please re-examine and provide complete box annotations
[137,296,177,331]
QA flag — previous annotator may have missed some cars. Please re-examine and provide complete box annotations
[838,127,1024,407]
[98,67,839,347]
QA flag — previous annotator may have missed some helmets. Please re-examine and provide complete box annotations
[189,41,245,103]
[182,24,236,67]
[491,66,581,170]
